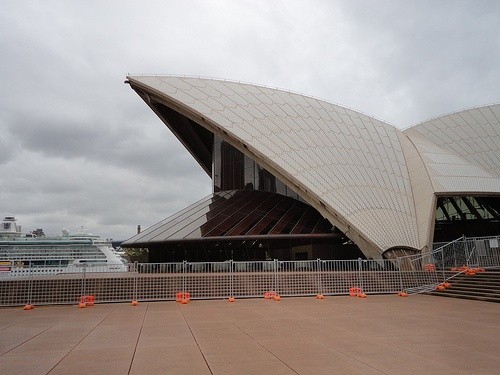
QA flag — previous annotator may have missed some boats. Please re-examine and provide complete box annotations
[0,228,128,275]
